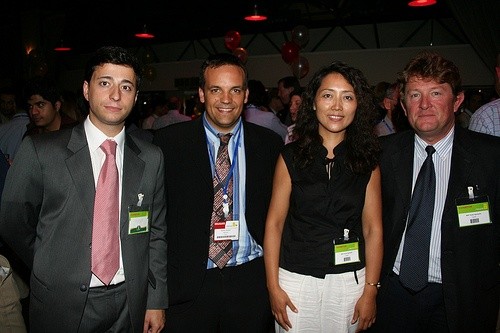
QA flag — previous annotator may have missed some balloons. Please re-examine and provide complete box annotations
[232,47,250,66]
[19,39,57,80]
[293,56,309,81]
[224,29,240,51]
[281,40,300,66]
[291,25,310,49]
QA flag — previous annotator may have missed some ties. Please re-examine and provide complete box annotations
[398,145,436,292]
[91,139,120,287]
[207,133,233,269]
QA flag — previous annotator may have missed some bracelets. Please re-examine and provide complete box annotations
[365,281,381,290]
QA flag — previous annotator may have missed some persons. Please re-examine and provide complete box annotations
[0,254,29,333]
[459,92,485,130]
[0,83,80,202]
[0,47,168,333]
[374,53,500,333]
[263,62,383,333]
[392,105,413,134]
[243,75,306,144]
[467,50,500,137]
[142,93,169,129]
[375,83,403,137]
[152,94,192,130]
[372,81,391,122]
[151,56,283,333]
[192,99,204,120]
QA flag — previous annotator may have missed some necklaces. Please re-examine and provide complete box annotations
[204,122,242,242]
[383,118,394,134]
